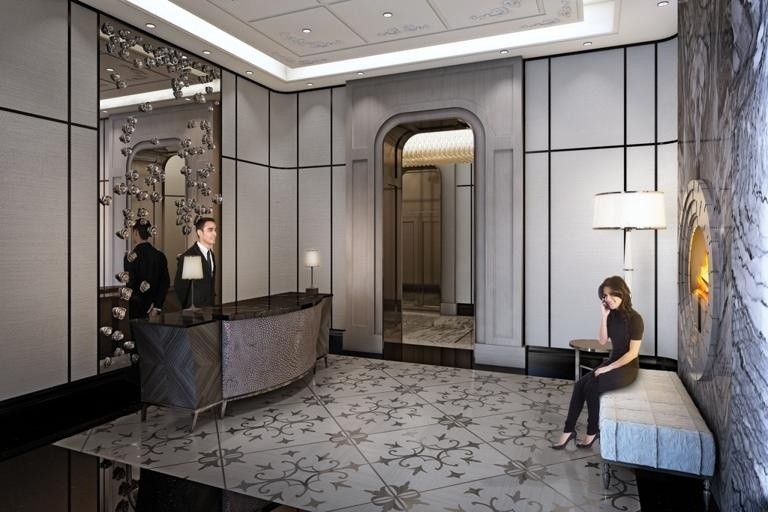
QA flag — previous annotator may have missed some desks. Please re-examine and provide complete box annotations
[132,292,333,432]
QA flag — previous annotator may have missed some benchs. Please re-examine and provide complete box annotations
[598,368,717,508]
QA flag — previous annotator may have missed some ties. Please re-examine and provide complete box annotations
[207,250,212,278]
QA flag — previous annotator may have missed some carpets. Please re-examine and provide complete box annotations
[51,354,643,511]
[401,311,473,351]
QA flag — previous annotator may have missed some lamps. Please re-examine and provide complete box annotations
[303,251,321,296]
[592,190,667,299]
[181,255,205,317]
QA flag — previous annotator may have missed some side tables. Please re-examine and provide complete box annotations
[570,339,613,383]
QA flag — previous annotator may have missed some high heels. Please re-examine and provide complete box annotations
[552,429,600,449]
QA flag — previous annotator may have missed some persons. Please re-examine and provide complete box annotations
[175,217,217,308]
[124,218,171,364]
[552,275,643,448]
